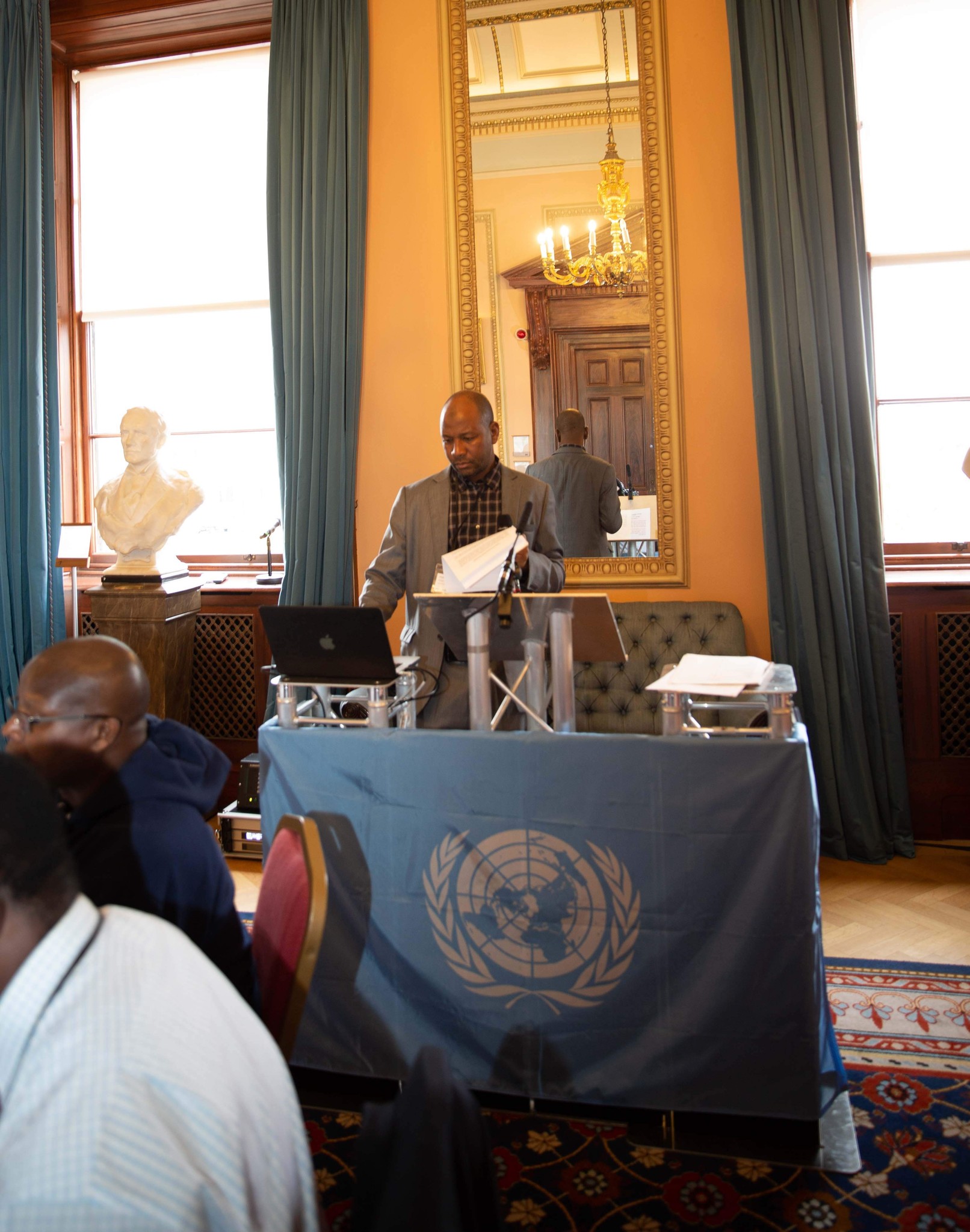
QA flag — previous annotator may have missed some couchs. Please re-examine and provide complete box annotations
[546,602,769,737]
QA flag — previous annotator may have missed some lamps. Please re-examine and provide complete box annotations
[537,0,648,300]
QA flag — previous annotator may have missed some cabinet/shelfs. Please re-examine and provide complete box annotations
[885,569,970,840]
[61,571,285,797]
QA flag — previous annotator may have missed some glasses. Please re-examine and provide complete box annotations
[7,697,123,735]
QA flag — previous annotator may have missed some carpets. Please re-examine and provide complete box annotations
[238,912,970,1232]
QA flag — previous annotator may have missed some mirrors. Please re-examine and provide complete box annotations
[439,0,690,590]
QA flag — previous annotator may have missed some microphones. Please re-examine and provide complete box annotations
[498,512,512,532]
[498,501,533,590]
[260,519,281,539]
[626,464,633,500]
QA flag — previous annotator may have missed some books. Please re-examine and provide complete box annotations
[646,652,774,696]
[444,522,528,596]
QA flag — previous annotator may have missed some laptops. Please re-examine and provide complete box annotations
[258,605,421,678]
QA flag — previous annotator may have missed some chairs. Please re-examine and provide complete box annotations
[248,813,329,1064]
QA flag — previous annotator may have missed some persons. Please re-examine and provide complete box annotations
[94,409,206,564]
[525,408,623,558]
[0,743,319,1232]
[0,634,263,1018]
[359,389,567,730]
